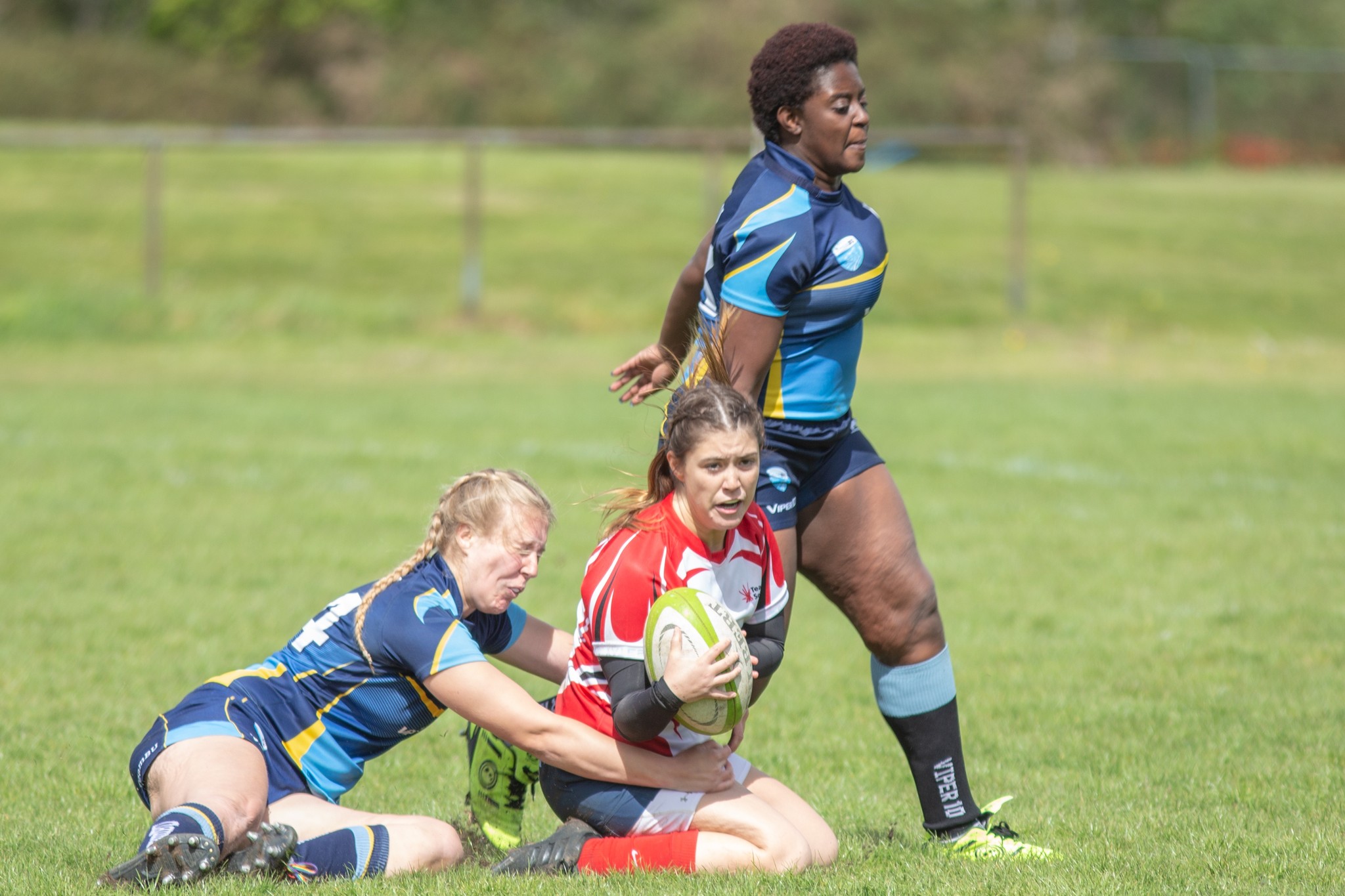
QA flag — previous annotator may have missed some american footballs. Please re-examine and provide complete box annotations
[646,588,754,738]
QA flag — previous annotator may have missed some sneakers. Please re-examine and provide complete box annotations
[460,720,539,854]
[929,794,1061,861]
[223,821,318,885]
[487,817,601,875]
[105,832,220,888]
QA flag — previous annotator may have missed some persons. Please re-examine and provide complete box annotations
[463,22,1070,855]
[99,467,749,889]
[489,383,839,875]
